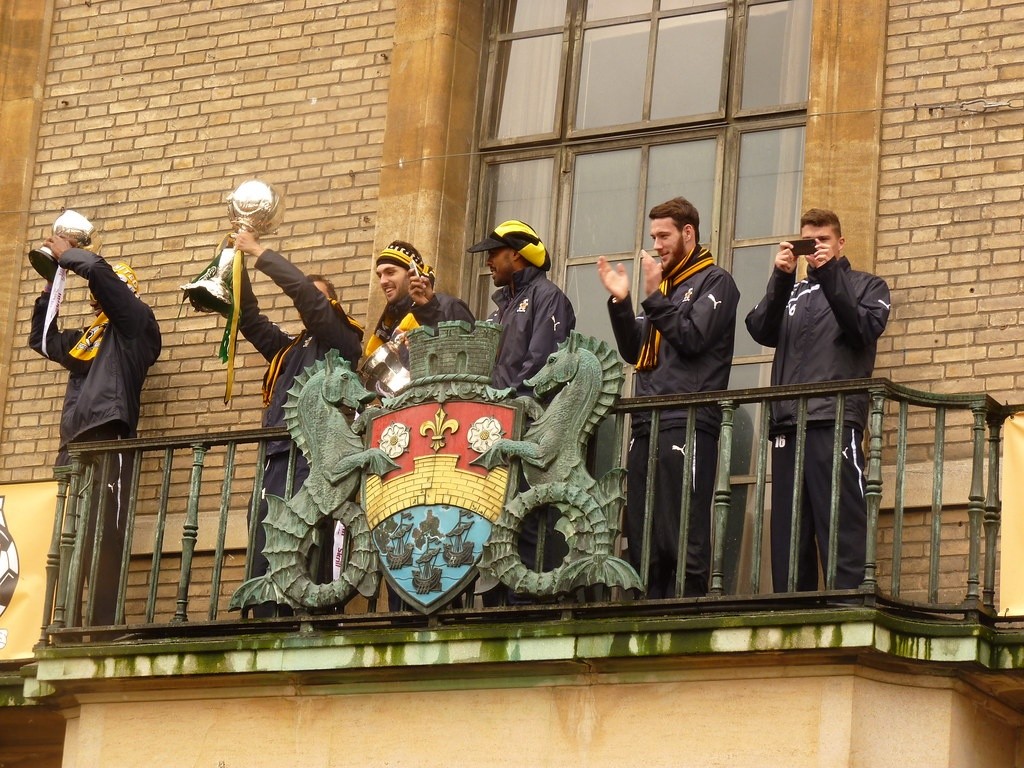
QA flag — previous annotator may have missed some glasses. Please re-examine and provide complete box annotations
[89,292,99,301]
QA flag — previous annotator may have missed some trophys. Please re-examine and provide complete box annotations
[359,332,411,397]
[180,178,284,321]
[28,209,103,282]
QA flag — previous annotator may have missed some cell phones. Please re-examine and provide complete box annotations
[784,239,816,256]
[409,261,423,283]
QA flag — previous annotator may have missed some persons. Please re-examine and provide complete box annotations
[229,232,364,632]
[743,209,890,594]
[597,196,741,600]
[409,218,577,604]
[361,239,476,628]
[27,236,162,644]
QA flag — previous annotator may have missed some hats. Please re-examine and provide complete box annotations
[376,241,425,276]
[465,221,552,272]
[110,263,139,294]
